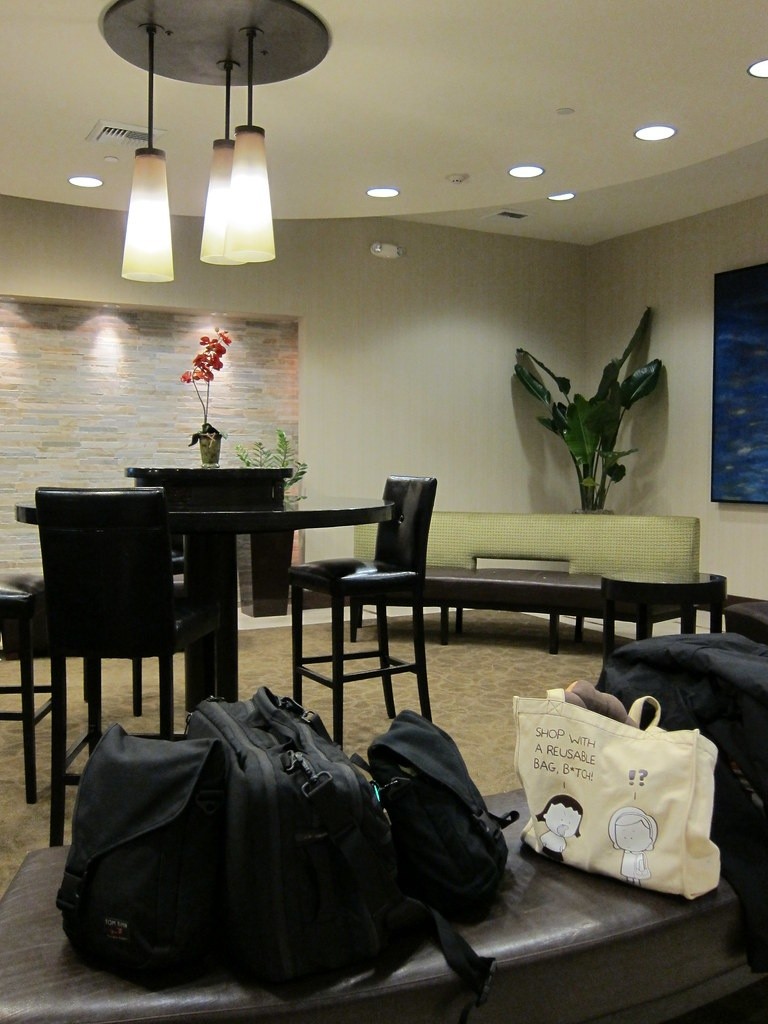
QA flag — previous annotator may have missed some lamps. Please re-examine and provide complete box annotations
[101,0,329,284]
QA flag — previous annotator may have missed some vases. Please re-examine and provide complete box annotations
[198,437,221,468]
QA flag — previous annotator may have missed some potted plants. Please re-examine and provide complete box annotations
[234,430,308,618]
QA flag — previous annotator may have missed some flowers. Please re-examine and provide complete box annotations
[181,326,232,447]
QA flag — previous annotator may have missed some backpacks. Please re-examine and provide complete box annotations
[349,711,521,926]
[594,632,768,974]
[55,724,227,992]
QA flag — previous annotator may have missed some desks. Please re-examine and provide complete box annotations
[16,499,397,722]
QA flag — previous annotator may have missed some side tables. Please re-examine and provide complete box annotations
[601,570,729,661]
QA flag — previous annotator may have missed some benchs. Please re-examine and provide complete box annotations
[0,784,768,1024]
[347,510,699,655]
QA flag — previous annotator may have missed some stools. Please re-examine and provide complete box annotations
[35,487,209,844]
[0,573,58,804]
[289,476,439,753]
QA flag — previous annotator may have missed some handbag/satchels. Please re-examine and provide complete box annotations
[513,688,720,899]
[182,686,498,1024]
